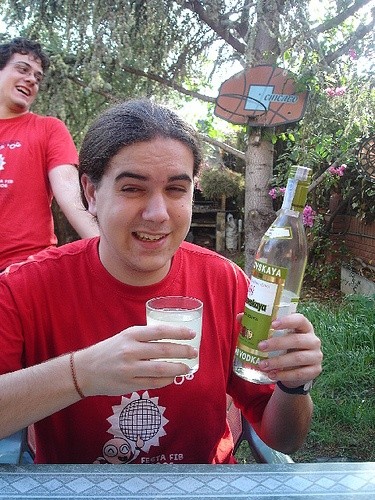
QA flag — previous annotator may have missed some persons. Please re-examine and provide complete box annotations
[1,95,323,464]
[0,37,104,271]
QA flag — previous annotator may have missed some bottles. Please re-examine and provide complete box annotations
[233,165,313,384]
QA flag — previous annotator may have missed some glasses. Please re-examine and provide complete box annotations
[7,61,42,84]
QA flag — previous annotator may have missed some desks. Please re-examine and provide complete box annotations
[0,462,375,500]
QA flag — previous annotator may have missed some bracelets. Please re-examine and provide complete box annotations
[69,352,86,403]
[276,378,316,395]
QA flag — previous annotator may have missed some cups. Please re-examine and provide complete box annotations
[145,296,203,375]
